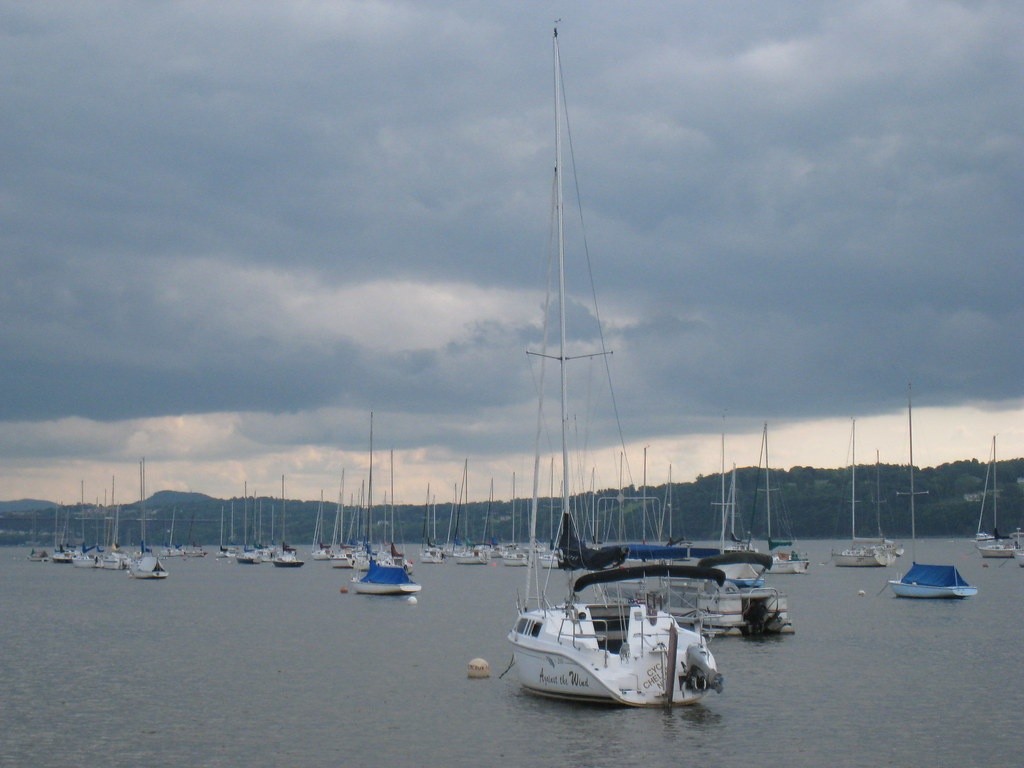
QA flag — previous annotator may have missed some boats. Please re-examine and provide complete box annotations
[1014,552,1024,569]
[346,541,424,597]
[26,548,50,563]
[976,533,994,542]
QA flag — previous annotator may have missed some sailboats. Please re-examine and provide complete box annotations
[420,23,795,645]
[751,422,812,575]
[506,167,726,722]
[310,411,415,575]
[976,434,1016,558]
[831,416,897,568]
[886,379,980,600]
[53,457,207,588]
[852,449,905,558]
[215,473,305,568]
